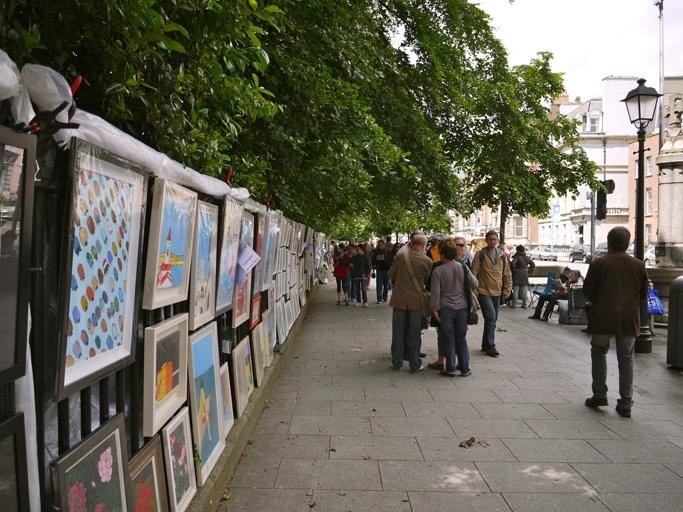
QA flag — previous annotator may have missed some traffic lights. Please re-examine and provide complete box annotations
[597,182,607,219]
[606,180,615,193]
[579,236,583,245]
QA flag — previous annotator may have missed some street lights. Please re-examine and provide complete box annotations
[620,77,665,352]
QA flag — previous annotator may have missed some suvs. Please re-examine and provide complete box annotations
[595,242,638,263]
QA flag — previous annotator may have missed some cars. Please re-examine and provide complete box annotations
[642,246,657,267]
[503,244,531,259]
[567,243,594,263]
[529,246,559,262]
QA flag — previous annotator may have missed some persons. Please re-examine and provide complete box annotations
[582,227,649,418]
[331,230,513,377]
[529,270,583,321]
[507,245,535,308]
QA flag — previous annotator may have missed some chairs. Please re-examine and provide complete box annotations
[529,271,555,309]
[568,285,589,326]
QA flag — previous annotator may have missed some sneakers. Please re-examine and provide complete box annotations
[615,398,632,418]
[440,365,460,377]
[585,396,608,408]
[337,286,393,308]
[427,362,444,370]
[461,368,471,377]
[482,345,499,357]
[393,352,427,373]
[500,301,551,322]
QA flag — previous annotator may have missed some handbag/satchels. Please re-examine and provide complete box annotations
[647,288,664,316]
[467,311,479,325]
[420,289,432,319]
